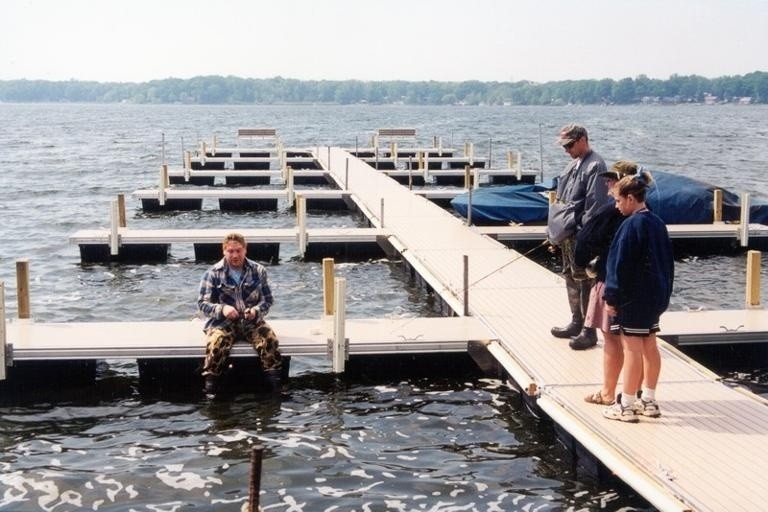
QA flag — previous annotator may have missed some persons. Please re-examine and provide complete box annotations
[548,123,608,351]
[600,165,675,423]
[196,231,283,408]
[569,159,658,406]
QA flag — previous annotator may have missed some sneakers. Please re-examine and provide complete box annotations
[603,393,640,422]
[569,326,597,348]
[635,390,661,416]
[551,319,583,337]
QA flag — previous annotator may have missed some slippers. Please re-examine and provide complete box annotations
[585,390,615,405]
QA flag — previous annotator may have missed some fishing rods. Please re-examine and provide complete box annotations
[398,241,548,332]
[249,255,275,312]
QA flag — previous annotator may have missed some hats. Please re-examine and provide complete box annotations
[598,161,637,180]
[557,125,578,145]
